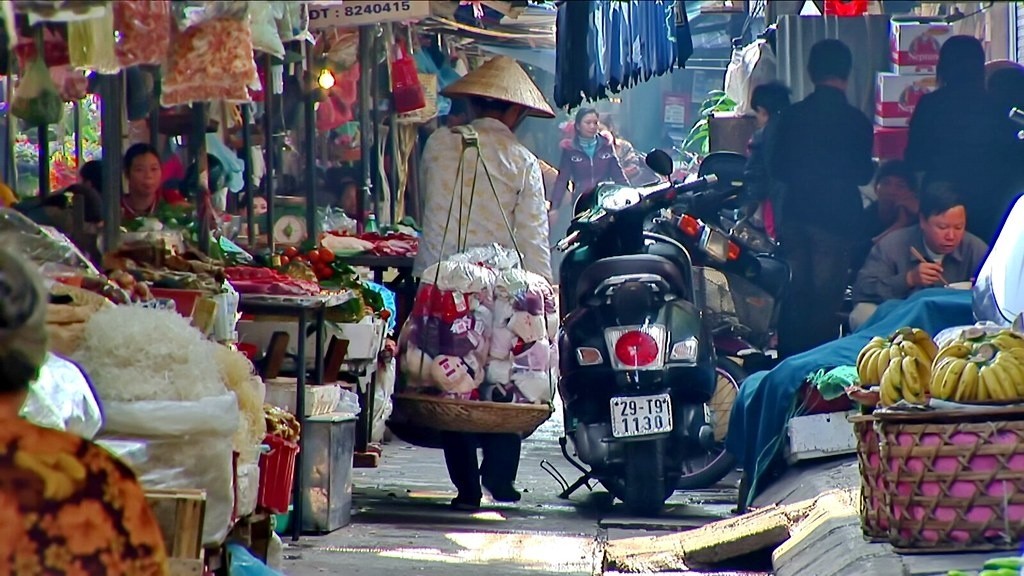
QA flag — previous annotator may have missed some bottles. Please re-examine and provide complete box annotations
[363,214,378,233]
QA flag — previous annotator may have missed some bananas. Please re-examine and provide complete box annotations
[857,328,1024,406]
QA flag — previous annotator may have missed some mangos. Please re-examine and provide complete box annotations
[947,557,1023,576]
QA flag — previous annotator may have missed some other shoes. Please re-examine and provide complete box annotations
[452,489,480,509]
[481,479,521,502]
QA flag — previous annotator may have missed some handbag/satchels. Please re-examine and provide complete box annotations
[11,25,64,124]
[391,38,426,112]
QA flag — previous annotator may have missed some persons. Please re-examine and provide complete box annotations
[0,243,169,576]
[546,108,640,223]
[79,142,179,222]
[846,36,1024,334]
[760,38,875,361]
[236,163,357,220]
[729,82,790,249]
[411,54,556,512]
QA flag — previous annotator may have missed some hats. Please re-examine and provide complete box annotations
[437,55,557,118]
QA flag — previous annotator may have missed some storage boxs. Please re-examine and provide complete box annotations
[783,408,866,464]
[869,21,957,161]
[253,434,300,513]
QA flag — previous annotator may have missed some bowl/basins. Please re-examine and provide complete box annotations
[944,281,972,290]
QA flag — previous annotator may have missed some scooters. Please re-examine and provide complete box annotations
[632,147,802,493]
[539,147,720,515]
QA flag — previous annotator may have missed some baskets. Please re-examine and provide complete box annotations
[854,421,1024,550]
[391,394,553,434]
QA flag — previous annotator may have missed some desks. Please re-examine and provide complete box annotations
[327,254,414,289]
[133,284,356,543]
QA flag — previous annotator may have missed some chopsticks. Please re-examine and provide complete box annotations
[910,247,949,286]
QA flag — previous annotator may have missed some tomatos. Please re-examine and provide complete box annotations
[280,246,334,278]
